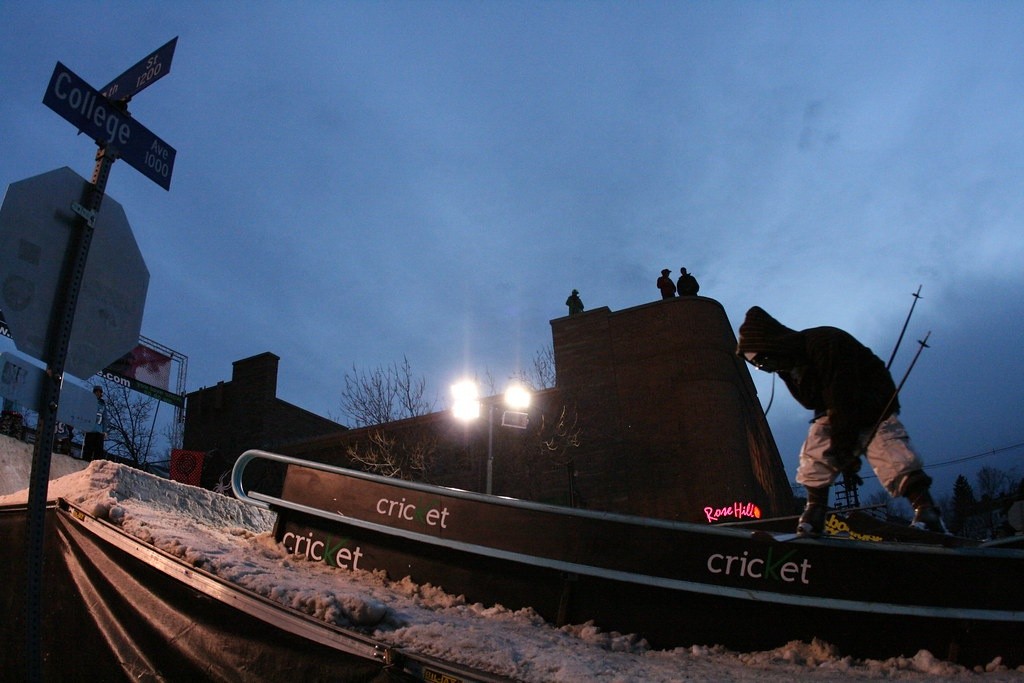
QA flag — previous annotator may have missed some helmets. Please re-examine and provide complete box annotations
[572,289,579,294]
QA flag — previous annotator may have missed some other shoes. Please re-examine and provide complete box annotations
[847,480,990,546]
[746,483,852,547]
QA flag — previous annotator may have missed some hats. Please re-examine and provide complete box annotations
[660,268,672,274]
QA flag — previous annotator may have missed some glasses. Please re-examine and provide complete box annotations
[742,352,766,367]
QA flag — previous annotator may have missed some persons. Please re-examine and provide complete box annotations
[656,269,677,300]
[565,289,585,316]
[80,385,109,462]
[735,305,949,540]
[676,267,699,296]
[53,420,74,457]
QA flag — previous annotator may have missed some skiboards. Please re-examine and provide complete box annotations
[845,511,983,544]
[751,523,850,544]
